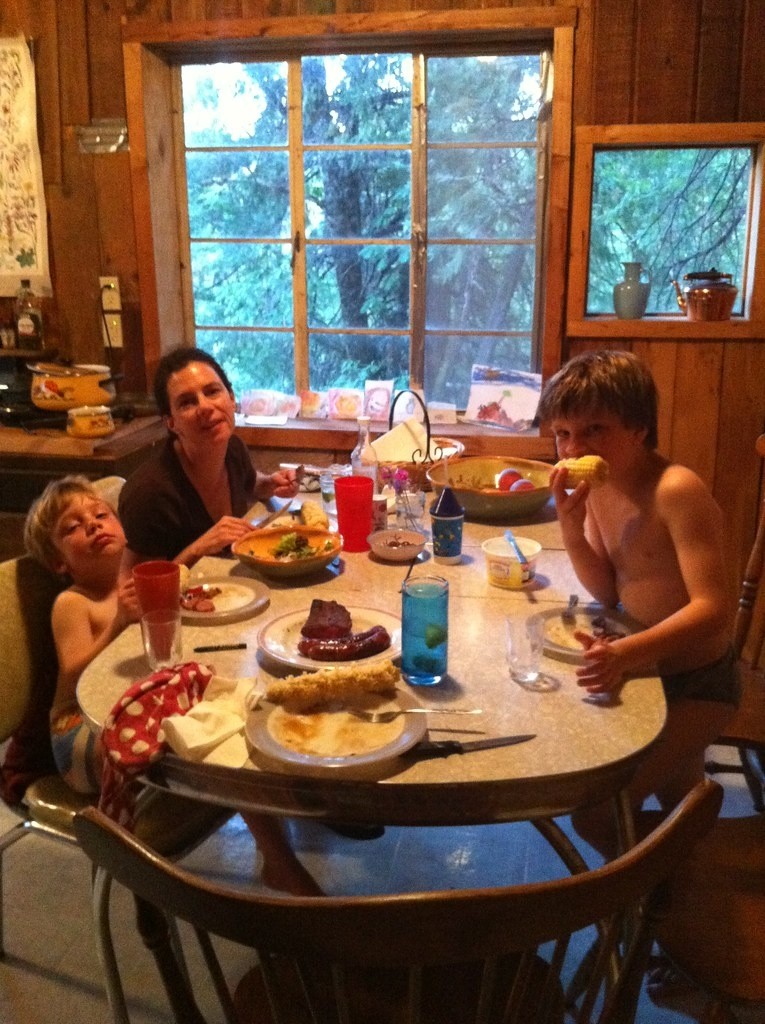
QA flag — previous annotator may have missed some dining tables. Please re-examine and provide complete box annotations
[77,467,669,1024]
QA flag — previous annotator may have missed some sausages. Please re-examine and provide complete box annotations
[298,624,392,662]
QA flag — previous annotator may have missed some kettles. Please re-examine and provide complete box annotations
[671,267,738,322]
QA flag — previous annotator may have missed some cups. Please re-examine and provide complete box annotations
[504,609,544,684]
[140,610,184,671]
[400,575,449,686]
[132,561,180,662]
[320,472,388,553]
[429,505,465,565]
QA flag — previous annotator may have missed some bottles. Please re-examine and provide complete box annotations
[351,417,379,493]
[14,280,41,350]
[614,262,650,320]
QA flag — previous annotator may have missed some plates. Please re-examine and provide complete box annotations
[245,688,426,769]
[256,604,401,670]
[180,575,271,621]
[524,607,647,659]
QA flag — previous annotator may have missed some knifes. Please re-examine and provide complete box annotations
[504,530,527,565]
[397,734,537,757]
[223,500,291,550]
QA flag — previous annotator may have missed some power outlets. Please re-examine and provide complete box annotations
[99,276,122,311]
[104,315,123,347]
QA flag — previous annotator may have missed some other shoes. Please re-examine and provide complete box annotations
[324,818,384,841]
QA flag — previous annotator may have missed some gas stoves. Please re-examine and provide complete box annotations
[0,395,131,433]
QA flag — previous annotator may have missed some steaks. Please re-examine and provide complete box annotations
[300,599,352,639]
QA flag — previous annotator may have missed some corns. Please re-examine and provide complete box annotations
[548,455,611,489]
[301,500,329,531]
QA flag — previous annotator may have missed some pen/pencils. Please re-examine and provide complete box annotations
[194,643,247,652]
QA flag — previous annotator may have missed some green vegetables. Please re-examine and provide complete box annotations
[273,532,336,559]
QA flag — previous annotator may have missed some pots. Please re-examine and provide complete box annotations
[30,361,124,411]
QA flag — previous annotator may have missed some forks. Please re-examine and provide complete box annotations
[347,704,482,724]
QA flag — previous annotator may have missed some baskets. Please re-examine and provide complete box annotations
[361,392,465,492]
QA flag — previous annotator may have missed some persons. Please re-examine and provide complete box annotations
[118,345,387,896]
[535,346,742,976]
[25,474,236,950]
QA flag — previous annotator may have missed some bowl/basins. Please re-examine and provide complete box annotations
[67,406,115,437]
[366,529,427,561]
[480,535,543,589]
[426,455,555,521]
[229,524,344,577]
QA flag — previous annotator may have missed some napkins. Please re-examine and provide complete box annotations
[159,675,262,769]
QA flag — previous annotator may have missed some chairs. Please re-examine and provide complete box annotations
[700,433,764,813]
[635,810,765,1024]
[74,779,723,1024]
[0,552,238,962]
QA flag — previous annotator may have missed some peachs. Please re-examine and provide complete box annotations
[496,469,534,491]
